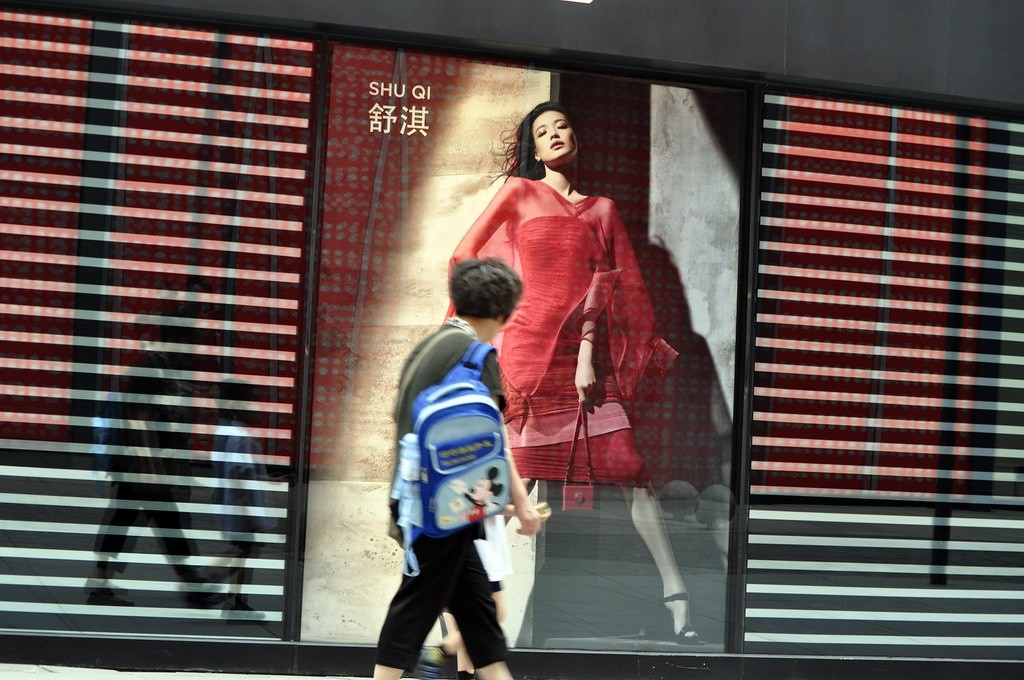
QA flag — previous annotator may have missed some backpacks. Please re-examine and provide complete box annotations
[390,343,514,577]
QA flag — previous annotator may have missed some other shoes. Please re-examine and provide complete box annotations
[419,647,477,680]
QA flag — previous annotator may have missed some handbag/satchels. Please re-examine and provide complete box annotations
[562,483,595,510]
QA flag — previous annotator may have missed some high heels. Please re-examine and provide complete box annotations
[660,590,704,644]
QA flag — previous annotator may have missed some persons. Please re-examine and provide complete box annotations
[435,101,703,645]
[372,258,543,680]
[419,476,552,680]
[79,310,274,621]
